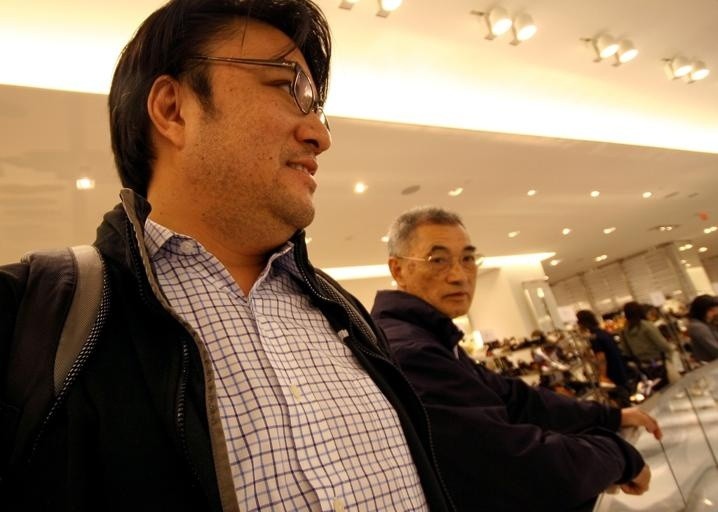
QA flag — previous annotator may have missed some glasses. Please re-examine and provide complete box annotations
[184,54,330,131]
[390,253,487,270]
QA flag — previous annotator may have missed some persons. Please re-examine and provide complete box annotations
[528,330,575,398]
[687,293,717,368]
[618,299,675,393]
[575,308,641,408]
[639,304,685,379]
[370,204,665,512]
[0,0,456,512]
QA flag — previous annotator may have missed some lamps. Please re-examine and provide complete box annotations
[473,2,541,48]
[664,51,713,89]
[587,29,642,67]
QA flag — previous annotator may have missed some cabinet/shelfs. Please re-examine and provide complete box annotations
[471,331,597,402]
